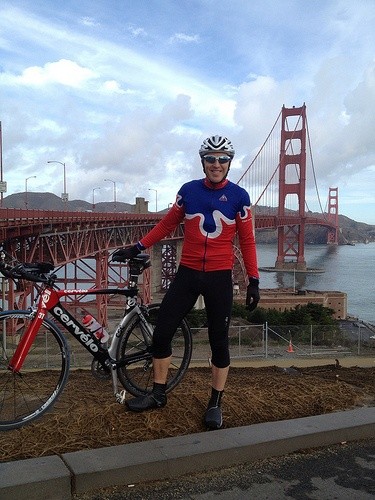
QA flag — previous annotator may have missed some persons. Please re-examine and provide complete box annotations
[112,135,260,430]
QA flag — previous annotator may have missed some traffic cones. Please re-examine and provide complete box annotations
[286,340,295,353]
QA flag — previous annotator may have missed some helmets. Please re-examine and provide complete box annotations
[199,135,235,160]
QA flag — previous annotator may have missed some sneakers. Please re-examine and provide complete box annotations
[204,398,223,430]
[125,391,167,413]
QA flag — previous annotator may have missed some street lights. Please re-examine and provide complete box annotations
[93,187,101,203]
[47,161,67,210]
[25,176,37,209]
[104,178,116,202]
[149,188,157,212]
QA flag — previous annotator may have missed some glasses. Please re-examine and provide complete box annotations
[203,154,232,164]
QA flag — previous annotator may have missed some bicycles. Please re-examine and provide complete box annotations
[0,247,193,431]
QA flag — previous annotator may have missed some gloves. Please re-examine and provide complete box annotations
[245,277,260,312]
[112,240,146,263]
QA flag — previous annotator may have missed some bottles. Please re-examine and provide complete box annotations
[81,314,109,343]
